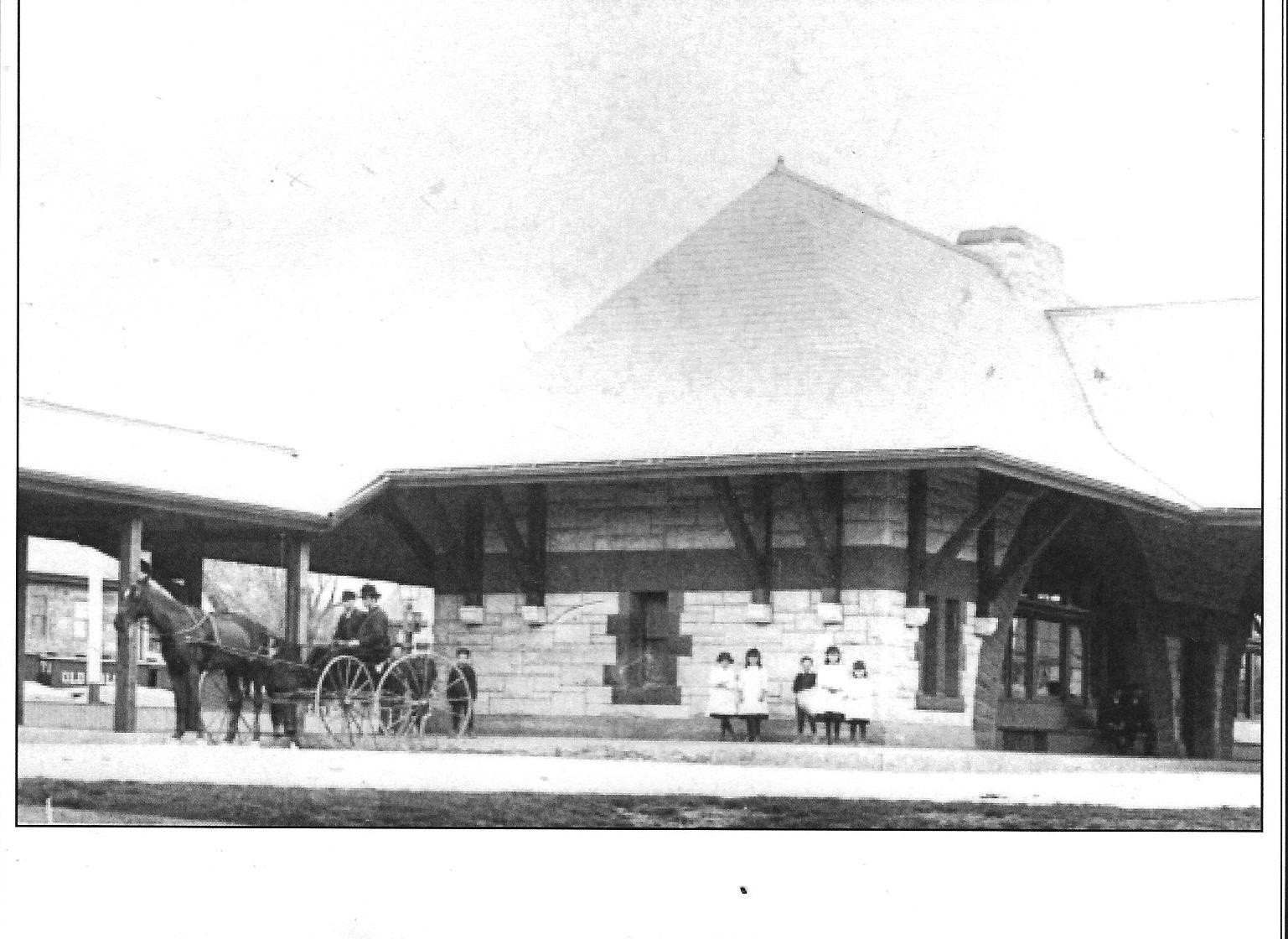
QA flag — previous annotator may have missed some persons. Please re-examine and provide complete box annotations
[706,646,870,746]
[1097,684,1159,757]
[302,586,477,738]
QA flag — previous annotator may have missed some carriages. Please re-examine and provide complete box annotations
[112,574,476,753]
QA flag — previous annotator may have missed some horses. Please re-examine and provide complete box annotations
[112,571,303,750]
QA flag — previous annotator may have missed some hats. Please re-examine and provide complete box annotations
[359,584,382,598]
[341,591,356,602]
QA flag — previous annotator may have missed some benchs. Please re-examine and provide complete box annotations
[1074,728,1148,756]
